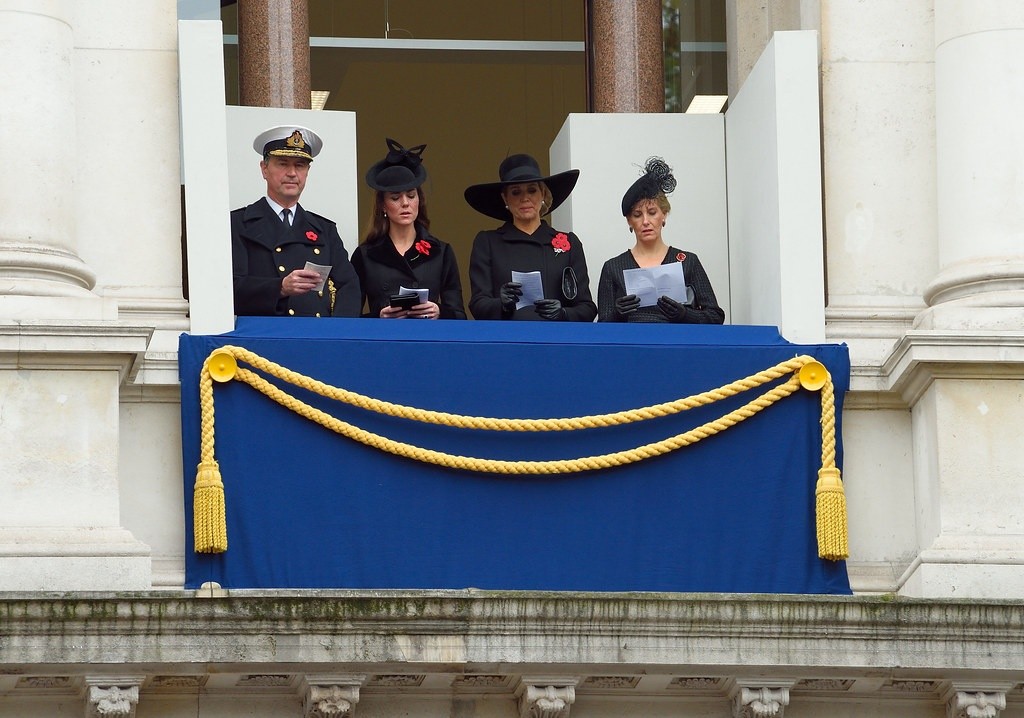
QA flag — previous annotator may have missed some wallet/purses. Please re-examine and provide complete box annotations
[389,292,419,310]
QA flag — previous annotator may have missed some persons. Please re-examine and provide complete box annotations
[230,125,360,317]
[464,154,597,322]
[351,138,468,319]
[597,159,725,325]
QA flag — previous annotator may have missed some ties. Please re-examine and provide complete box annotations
[282,210,291,227]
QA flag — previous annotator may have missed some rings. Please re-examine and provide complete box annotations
[423,314,428,318]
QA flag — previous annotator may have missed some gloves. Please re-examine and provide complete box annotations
[534,297,562,321]
[614,294,640,321]
[656,294,687,322]
[500,281,524,312]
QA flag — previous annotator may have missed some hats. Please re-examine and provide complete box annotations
[463,154,579,221]
[622,157,678,217]
[367,137,427,192]
[253,125,323,162]
